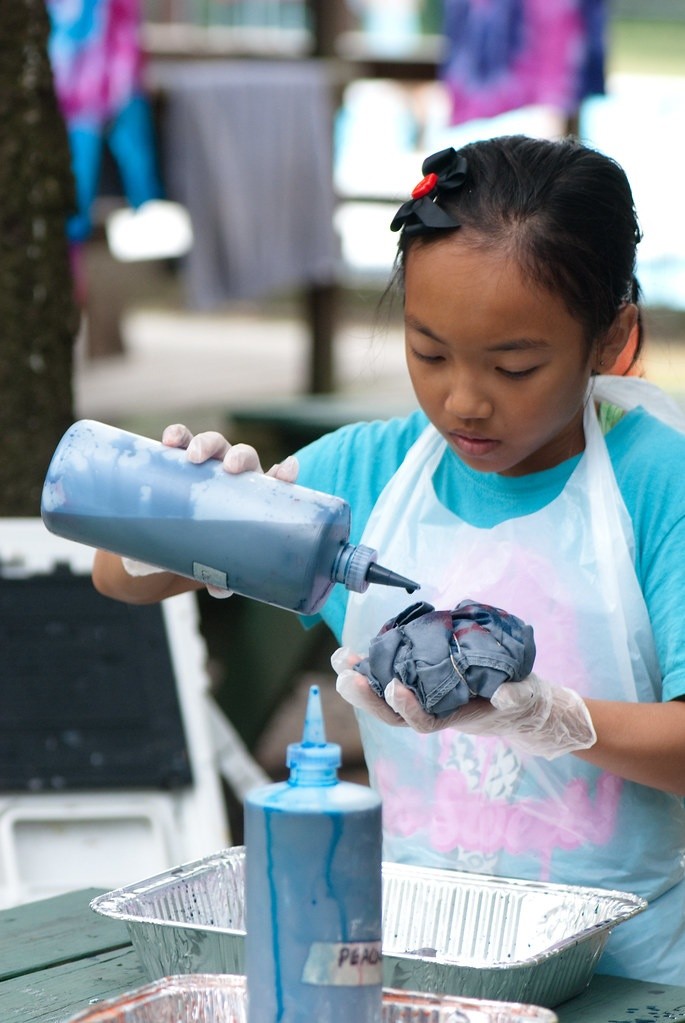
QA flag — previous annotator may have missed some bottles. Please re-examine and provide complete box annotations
[42,420,421,617]
[244,685,387,1023]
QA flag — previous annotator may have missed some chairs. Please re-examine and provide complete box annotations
[0,516,276,907]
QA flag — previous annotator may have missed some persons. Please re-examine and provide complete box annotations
[92,131,685,990]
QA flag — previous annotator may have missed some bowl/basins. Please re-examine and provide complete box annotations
[89,837,649,1009]
[64,972,562,1023]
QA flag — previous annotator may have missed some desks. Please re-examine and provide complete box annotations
[0,887,685,1023]
[81,56,354,403]
